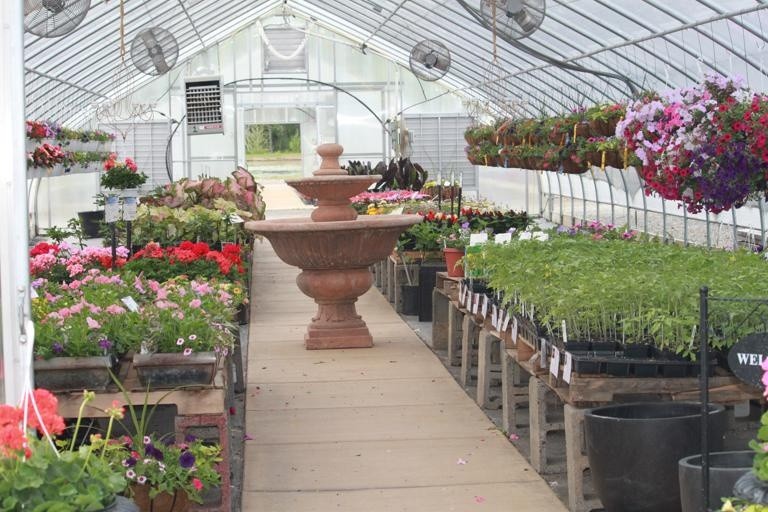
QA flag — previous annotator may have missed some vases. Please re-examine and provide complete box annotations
[733,470,768,504]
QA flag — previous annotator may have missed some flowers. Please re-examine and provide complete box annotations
[615,55,768,213]
[299,180,642,248]
[28,163,266,308]
[747,356,768,481]
[465,105,628,169]
[26,121,120,181]
[0,358,196,512]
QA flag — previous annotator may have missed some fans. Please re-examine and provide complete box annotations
[21,0,91,38]
[130,26,178,77]
[410,39,450,81]
[480,0,546,40]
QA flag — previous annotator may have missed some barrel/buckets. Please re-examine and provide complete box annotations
[100,187,139,223]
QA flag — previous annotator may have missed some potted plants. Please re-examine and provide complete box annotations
[129,292,228,388]
[459,233,768,378]
[440,238,468,277]
[99,154,148,198]
[24,291,129,391]
